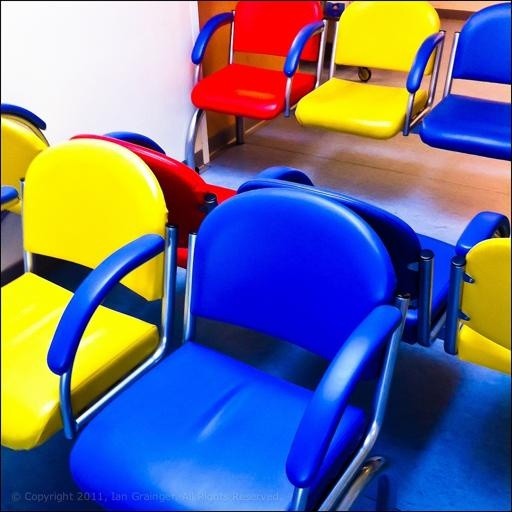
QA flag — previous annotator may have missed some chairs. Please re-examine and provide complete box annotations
[1,105,510,512]
